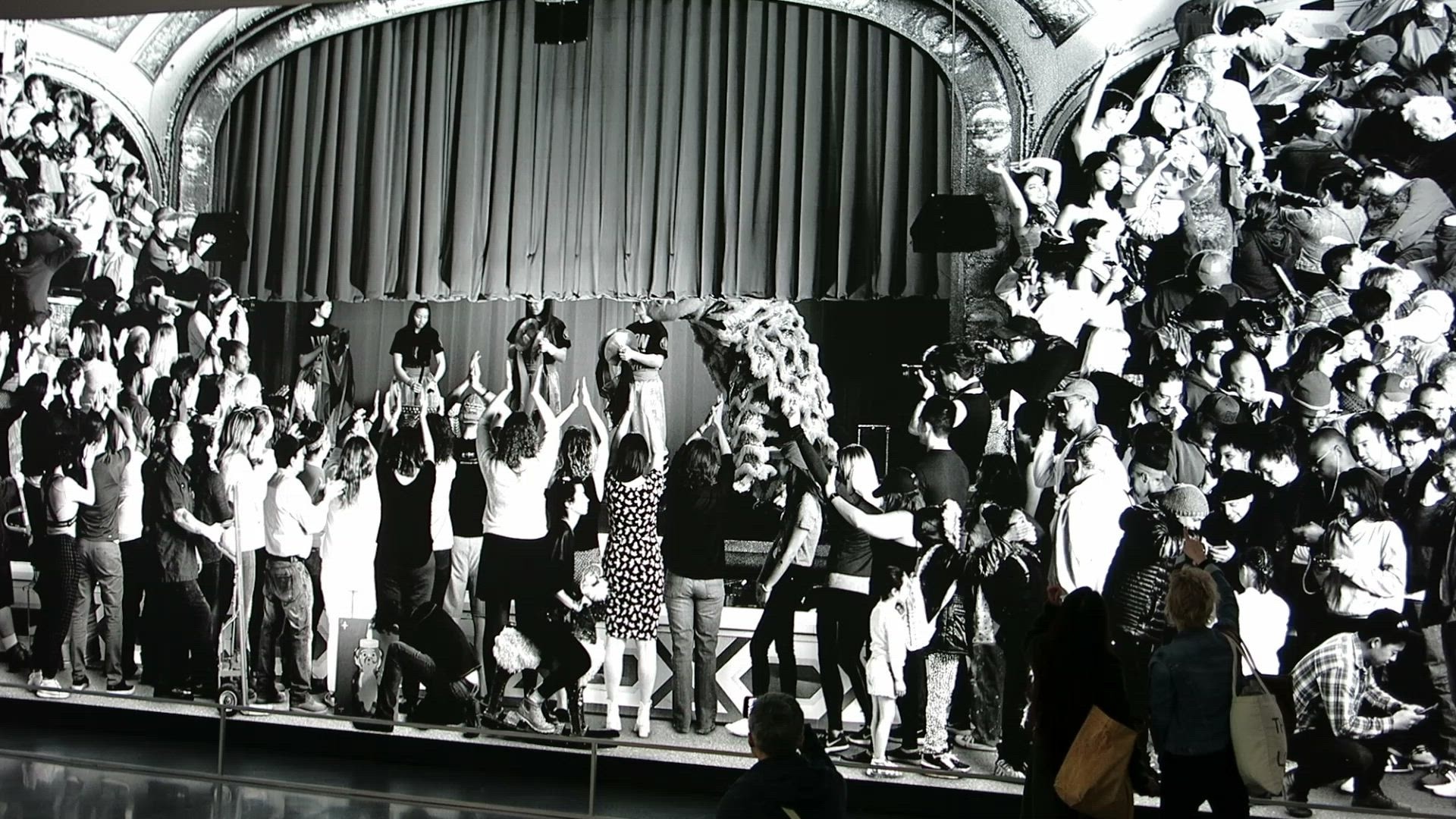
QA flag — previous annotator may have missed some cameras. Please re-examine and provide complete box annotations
[900,357,942,385]
[976,336,1004,356]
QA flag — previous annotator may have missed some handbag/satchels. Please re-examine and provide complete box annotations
[1215,626,1288,799]
[1052,702,1139,819]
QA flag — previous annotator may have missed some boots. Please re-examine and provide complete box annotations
[632,700,653,738]
[603,697,624,734]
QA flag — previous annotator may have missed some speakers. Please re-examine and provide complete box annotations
[190,211,250,262]
[533,0,589,46]
[911,195,998,254]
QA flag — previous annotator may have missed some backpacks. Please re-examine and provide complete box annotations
[893,544,960,654]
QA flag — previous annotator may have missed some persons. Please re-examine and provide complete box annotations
[787,0,1456,819]
[0,31,737,739]
[724,441,823,736]
[715,691,848,819]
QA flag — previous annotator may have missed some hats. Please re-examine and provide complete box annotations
[1365,607,1425,644]
[0,135,1456,551]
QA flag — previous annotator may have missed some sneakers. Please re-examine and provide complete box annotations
[27,669,44,693]
[1383,753,1414,774]
[72,674,91,691]
[289,692,329,715]
[1419,760,1456,799]
[105,678,136,695]
[514,696,555,734]
[34,678,72,699]
[1351,785,1411,813]
[1281,770,1312,819]
[671,714,1027,784]
[481,704,521,729]
[1410,745,1438,768]
[256,690,286,704]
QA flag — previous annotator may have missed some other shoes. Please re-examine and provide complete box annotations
[153,687,197,701]
[462,697,483,739]
[5,643,30,674]
[1339,776,1354,794]
[352,712,396,733]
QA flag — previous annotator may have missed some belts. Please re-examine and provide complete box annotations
[267,554,303,563]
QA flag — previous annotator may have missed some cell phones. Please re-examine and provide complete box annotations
[1418,702,1440,714]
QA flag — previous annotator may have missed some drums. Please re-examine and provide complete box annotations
[393,403,437,424]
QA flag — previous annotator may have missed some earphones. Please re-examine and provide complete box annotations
[1337,451,1340,457]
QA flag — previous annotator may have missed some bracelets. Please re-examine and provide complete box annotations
[697,427,703,435]
[849,489,855,496]
[829,493,839,502]
[761,584,773,592]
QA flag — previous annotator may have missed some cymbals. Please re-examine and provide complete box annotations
[604,328,639,366]
[529,329,544,368]
[515,318,539,352]
[328,327,351,362]
[597,327,625,359]
